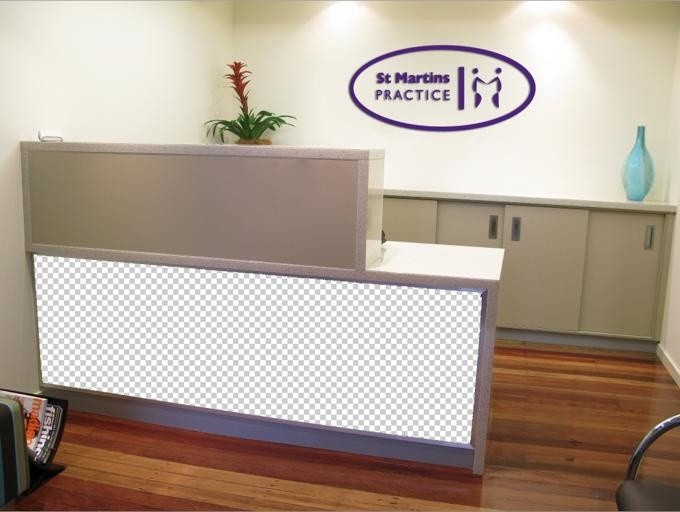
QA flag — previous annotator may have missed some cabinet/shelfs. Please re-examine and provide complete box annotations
[437,191,590,351]
[384,187,437,245]
[578,199,679,355]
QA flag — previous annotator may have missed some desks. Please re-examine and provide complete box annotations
[20,141,504,471]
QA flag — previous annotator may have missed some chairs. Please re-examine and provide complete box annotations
[614,413,680,512]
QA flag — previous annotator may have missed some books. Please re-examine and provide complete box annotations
[0,389,63,464]
